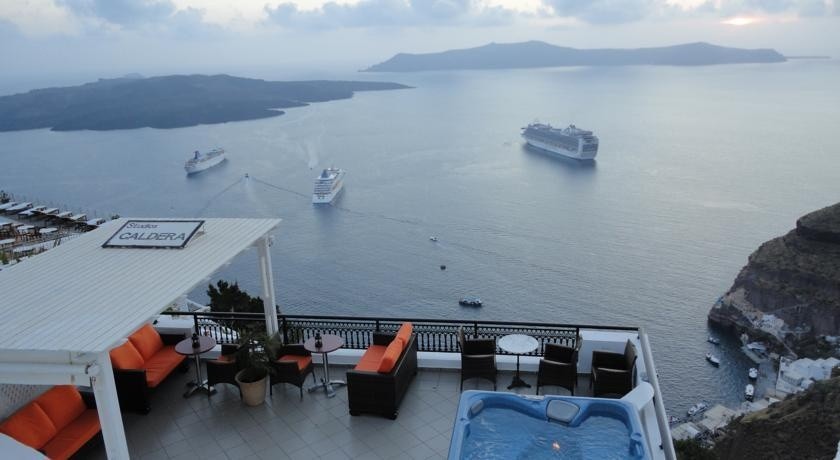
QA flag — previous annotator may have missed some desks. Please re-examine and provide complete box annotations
[304,334,348,398]
[497,333,539,389]
[175,334,218,398]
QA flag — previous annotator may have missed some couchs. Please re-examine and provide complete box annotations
[0,385,104,460]
[346,323,417,420]
[108,322,189,415]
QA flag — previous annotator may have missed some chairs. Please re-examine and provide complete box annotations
[207,341,249,402]
[456,325,498,394]
[589,338,638,399]
[536,337,583,397]
[0,200,107,272]
[261,332,317,400]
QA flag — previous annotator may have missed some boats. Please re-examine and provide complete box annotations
[707,335,722,345]
[308,164,343,206]
[183,146,226,175]
[687,400,707,414]
[707,353,720,365]
[744,385,755,399]
[749,367,759,379]
[521,121,598,165]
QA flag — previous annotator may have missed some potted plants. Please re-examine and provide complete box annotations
[234,325,285,407]
